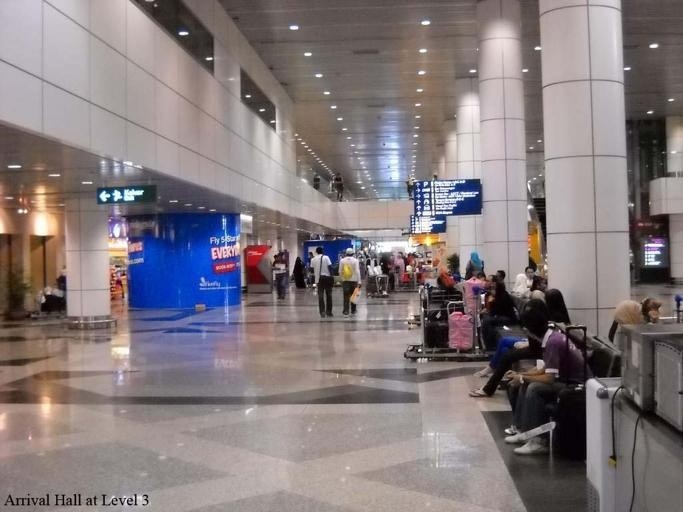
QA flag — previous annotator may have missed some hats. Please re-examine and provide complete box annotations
[346,248,354,255]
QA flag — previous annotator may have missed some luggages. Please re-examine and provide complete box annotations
[553,325,587,461]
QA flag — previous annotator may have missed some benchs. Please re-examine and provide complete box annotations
[477,292,622,450]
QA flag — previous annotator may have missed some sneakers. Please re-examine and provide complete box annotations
[322,310,356,319]
[505,425,547,455]
[475,368,490,376]
[469,388,489,397]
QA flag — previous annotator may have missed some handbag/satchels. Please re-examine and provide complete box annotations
[448,312,474,350]
[318,275,335,290]
[425,321,448,348]
[350,288,361,304]
[343,264,352,280]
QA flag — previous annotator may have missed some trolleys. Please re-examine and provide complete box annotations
[29,287,67,322]
[402,284,495,361]
[366,274,391,299]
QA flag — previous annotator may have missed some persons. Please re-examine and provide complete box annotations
[405,174,415,201]
[309,246,335,318]
[313,171,321,189]
[337,247,361,316]
[271,251,288,299]
[334,172,343,202]
[640,299,661,323]
[291,246,437,292]
[437,252,597,458]
